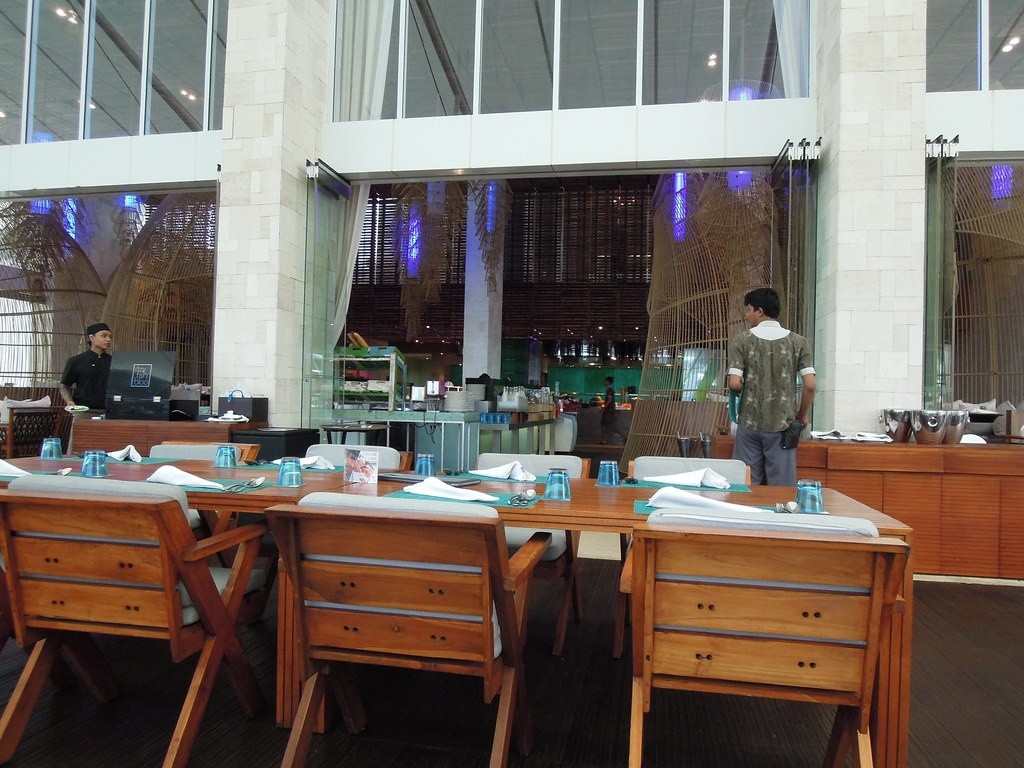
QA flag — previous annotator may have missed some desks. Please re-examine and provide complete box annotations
[320,425,390,446]
[336,410,565,472]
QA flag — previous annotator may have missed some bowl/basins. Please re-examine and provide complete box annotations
[942,410,969,444]
[911,411,947,444]
[884,409,913,442]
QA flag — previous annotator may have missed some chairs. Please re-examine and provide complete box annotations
[300,446,413,472]
[477,452,592,657]
[614,522,912,768]
[614,457,752,659]
[263,505,551,768]
[149,440,261,568]
[0,488,283,768]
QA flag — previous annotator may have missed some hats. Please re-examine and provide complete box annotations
[606,376,614,384]
[87,323,110,335]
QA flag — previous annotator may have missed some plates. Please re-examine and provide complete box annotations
[444,384,486,412]
[64,405,89,413]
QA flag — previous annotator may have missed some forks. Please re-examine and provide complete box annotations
[776,502,788,515]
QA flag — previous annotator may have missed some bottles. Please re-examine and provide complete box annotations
[501,386,526,402]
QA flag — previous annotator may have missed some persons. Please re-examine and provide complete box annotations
[728,288,817,486]
[477,373,494,401]
[599,376,628,446]
[60,323,113,454]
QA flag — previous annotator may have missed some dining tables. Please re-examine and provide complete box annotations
[0,456,402,730]
[295,470,914,768]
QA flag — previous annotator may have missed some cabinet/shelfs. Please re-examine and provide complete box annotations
[74,419,268,457]
[333,347,408,412]
[233,428,321,463]
[711,436,1024,581]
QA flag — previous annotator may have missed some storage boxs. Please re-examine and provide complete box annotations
[218,397,269,421]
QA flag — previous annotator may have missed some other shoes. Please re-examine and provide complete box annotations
[624,434,629,444]
[596,440,608,445]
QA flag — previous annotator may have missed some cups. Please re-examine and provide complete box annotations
[276,457,304,486]
[795,478,825,513]
[544,467,572,500]
[214,446,237,468]
[479,413,513,424]
[41,437,63,459]
[415,453,436,477]
[81,449,108,477]
[701,441,712,458]
[597,460,620,487]
[679,437,692,458]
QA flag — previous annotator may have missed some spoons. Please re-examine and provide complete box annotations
[508,489,536,505]
[221,476,265,492]
[45,468,72,476]
[786,501,799,513]
